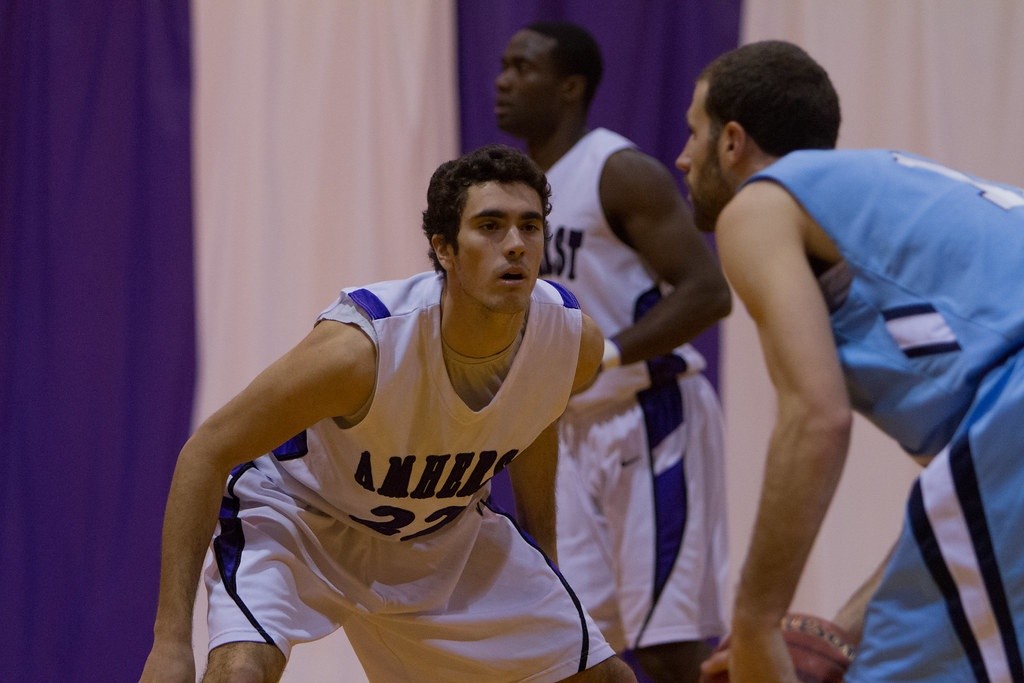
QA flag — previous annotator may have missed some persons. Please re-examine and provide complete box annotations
[489,6,731,683]
[136,144,644,683]
[672,38,1024,683]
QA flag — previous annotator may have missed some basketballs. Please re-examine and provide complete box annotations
[703,612,852,683]
